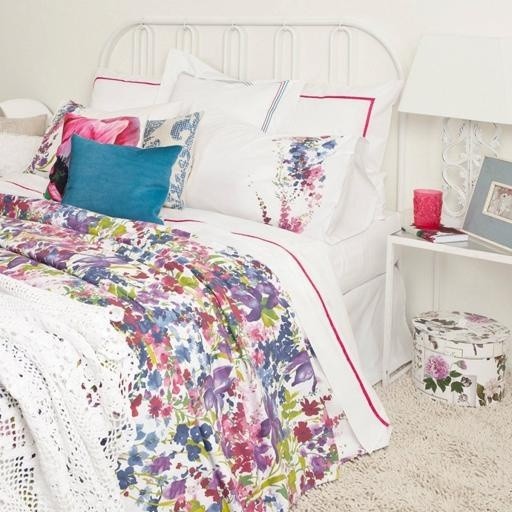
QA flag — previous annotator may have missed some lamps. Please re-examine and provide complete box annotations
[398,37,512,232]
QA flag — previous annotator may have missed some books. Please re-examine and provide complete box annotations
[403,224,469,244]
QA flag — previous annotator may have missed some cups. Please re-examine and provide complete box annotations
[413,188,443,230]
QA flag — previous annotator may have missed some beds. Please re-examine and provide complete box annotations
[1,19,406,512]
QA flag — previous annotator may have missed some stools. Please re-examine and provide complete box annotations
[409,307,512,406]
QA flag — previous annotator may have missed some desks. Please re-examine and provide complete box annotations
[382,229,512,389]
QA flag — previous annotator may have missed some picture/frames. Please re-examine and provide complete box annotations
[459,155,512,253]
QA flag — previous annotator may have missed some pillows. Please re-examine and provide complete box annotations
[22,98,195,179]
[2,96,53,115]
[0,113,49,138]
[41,111,142,203]
[184,131,361,239]
[158,51,307,134]
[63,134,182,226]
[3,131,44,178]
[284,80,395,218]
[89,73,159,106]
[142,116,203,209]
